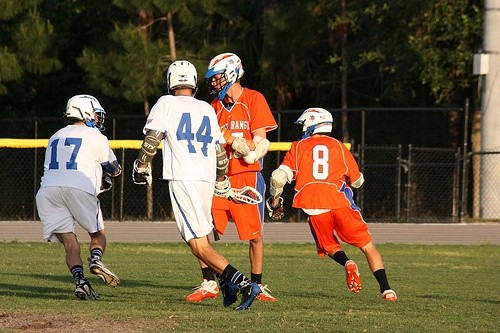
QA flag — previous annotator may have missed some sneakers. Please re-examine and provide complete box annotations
[75,279,100,301]
[87,257,120,289]
[219,281,239,307]
[381,289,397,302]
[233,280,262,311]
[345,259,362,293]
[185,279,220,304]
[254,284,277,303]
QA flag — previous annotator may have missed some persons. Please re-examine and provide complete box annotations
[184,54,276,302]
[35,95,122,300]
[266,108,397,301]
[133,60,263,310]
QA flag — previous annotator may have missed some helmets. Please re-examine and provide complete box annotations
[293,108,334,140]
[167,61,199,97]
[204,52,244,101]
[64,95,106,131]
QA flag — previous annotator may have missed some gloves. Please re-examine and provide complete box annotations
[213,175,232,197]
[100,175,112,193]
[132,159,151,185]
[105,164,122,177]
[266,196,285,220]
